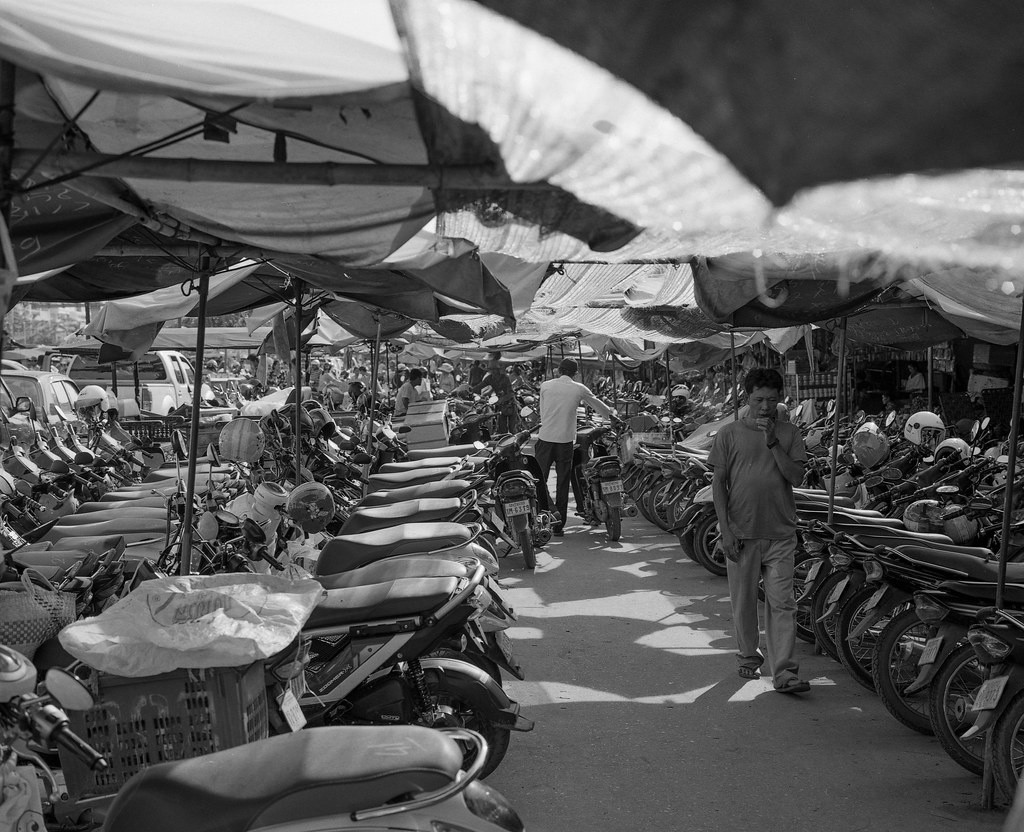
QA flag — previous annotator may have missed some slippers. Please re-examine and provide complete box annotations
[738,664,762,680]
[772,670,810,695]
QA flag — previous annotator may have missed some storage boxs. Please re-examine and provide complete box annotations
[56,658,270,803]
[403,399,451,449]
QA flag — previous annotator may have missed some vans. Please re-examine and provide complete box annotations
[0,369,80,438]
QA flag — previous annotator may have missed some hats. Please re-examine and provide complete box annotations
[396,363,454,373]
[560,357,578,372]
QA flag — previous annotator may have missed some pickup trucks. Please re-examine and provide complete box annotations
[66,349,217,438]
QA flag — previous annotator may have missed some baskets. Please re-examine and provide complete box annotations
[58,657,270,797]
[0,568,77,662]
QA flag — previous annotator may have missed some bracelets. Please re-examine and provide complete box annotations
[766,436,780,449]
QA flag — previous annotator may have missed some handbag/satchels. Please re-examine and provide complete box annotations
[499,402,516,416]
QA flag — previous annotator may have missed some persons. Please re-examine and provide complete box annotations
[200,351,534,437]
[533,356,625,538]
[881,390,899,414]
[706,367,814,695]
[904,360,926,397]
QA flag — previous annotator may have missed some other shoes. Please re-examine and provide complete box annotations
[553,529,564,537]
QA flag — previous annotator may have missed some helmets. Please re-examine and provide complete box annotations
[457,384,474,399]
[311,360,333,369]
[75,385,119,414]
[219,378,336,463]
[488,360,500,368]
[323,381,374,410]
[523,361,533,370]
[206,359,219,368]
[671,385,690,400]
[524,396,534,404]
[288,481,336,535]
[823,411,977,543]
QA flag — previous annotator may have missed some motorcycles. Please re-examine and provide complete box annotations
[570,421,638,541]
[0,373,541,832]
[473,421,561,568]
[575,375,1024,810]
